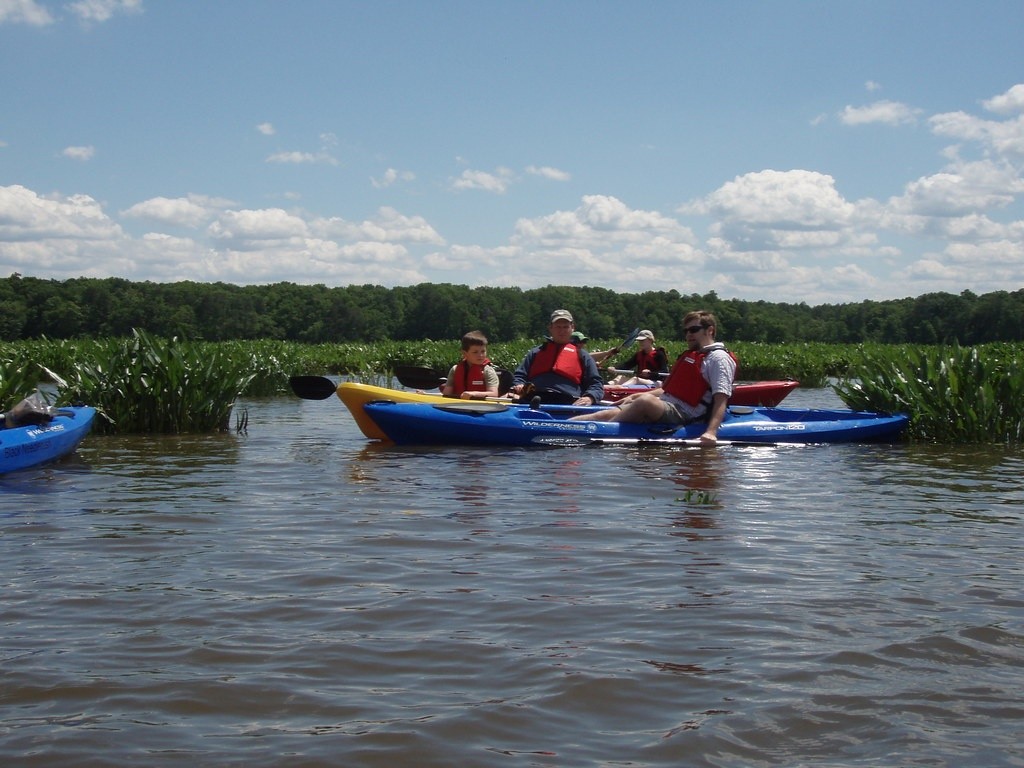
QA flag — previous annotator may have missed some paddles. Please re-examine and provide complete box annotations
[599,326,640,366]
[597,368,671,378]
[392,364,536,396]
[531,435,808,450]
[287,375,520,404]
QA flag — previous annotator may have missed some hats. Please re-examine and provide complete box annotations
[551,310,573,322]
[635,330,654,341]
[571,332,589,343]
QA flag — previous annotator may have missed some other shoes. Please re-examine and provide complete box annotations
[530,396,541,411]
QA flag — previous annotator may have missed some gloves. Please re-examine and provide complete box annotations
[604,348,620,357]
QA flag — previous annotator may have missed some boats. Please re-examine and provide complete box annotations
[0,405,98,478]
[359,399,910,448]
[598,378,799,410]
[335,380,519,448]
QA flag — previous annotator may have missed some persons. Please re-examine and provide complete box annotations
[514,311,606,405]
[417,331,499,402]
[604,330,669,387]
[571,332,619,367]
[566,311,738,449]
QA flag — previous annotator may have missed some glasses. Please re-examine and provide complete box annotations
[575,340,586,344]
[683,325,711,334]
